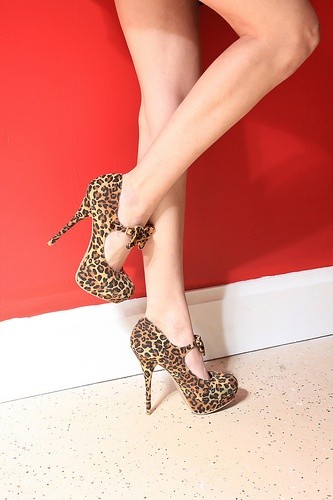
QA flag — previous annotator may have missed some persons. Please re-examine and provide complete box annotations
[46,0,321,418]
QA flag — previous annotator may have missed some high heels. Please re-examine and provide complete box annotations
[47,174,155,302]
[129,318,237,416]
